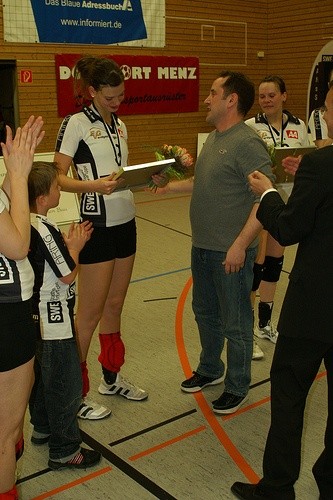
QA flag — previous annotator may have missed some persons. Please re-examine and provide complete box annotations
[180,69,276,415]
[0,114,48,500]
[308,107,332,148]
[231,82,333,500]
[53,56,170,421]
[27,160,102,469]
[243,75,310,361]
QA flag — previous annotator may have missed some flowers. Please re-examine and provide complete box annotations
[148,144,194,194]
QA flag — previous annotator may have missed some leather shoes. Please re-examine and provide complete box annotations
[231,481,295,500]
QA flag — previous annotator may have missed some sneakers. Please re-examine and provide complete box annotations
[98,372,149,401]
[212,392,249,414]
[48,446,101,471]
[31,425,51,445]
[181,371,225,392]
[254,320,278,344]
[76,398,112,420]
[252,341,264,359]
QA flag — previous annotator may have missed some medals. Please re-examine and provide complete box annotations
[91,100,123,173]
